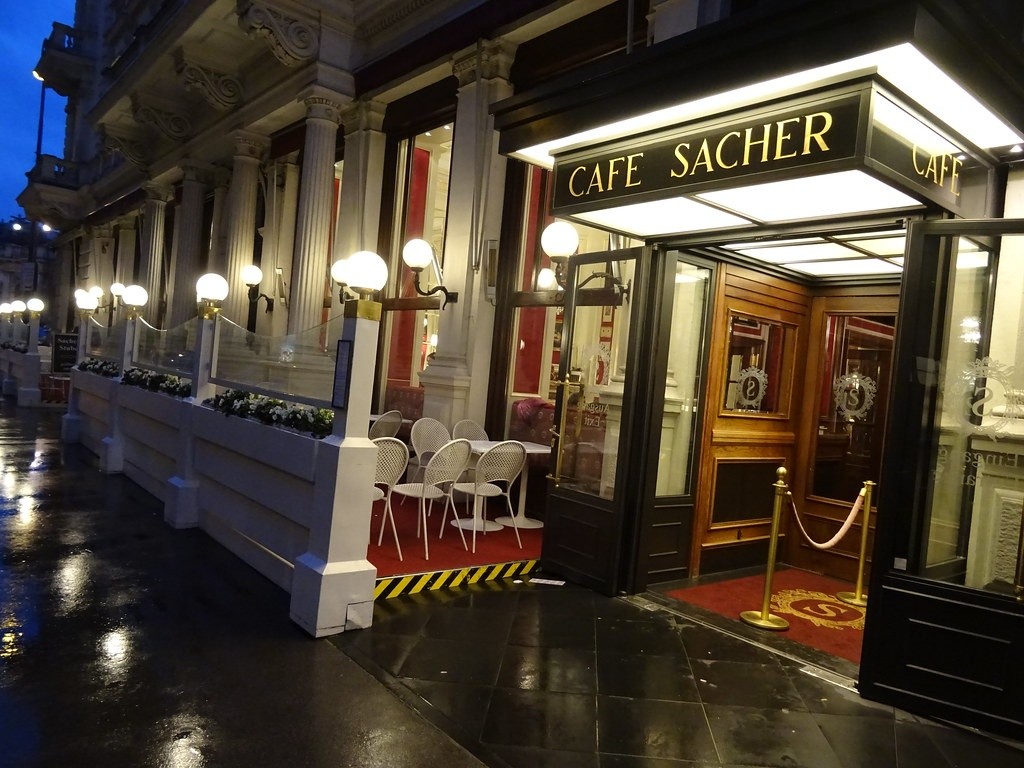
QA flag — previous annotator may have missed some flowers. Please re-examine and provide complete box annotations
[204,390,335,439]
[121,366,193,398]
[78,357,120,379]
[12,342,28,354]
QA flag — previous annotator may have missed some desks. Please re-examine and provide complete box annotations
[370,415,413,424]
[450,438,550,532]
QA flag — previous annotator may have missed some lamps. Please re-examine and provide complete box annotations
[122,285,148,310]
[89,286,112,309]
[0,303,12,320]
[74,288,88,300]
[11,301,27,318]
[539,221,632,307]
[332,259,355,304]
[195,273,229,308]
[110,283,126,309]
[76,294,100,314]
[347,249,390,299]
[240,264,275,313]
[27,298,44,318]
[402,239,459,310]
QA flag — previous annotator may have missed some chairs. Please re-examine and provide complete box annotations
[370,410,404,442]
[378,437,472,560]
[440,440,529,552]
[399,418,470,517]
[453,420,510,515]
[373,436,410,559]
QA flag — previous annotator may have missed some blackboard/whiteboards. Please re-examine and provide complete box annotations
[50,333,79,376]
